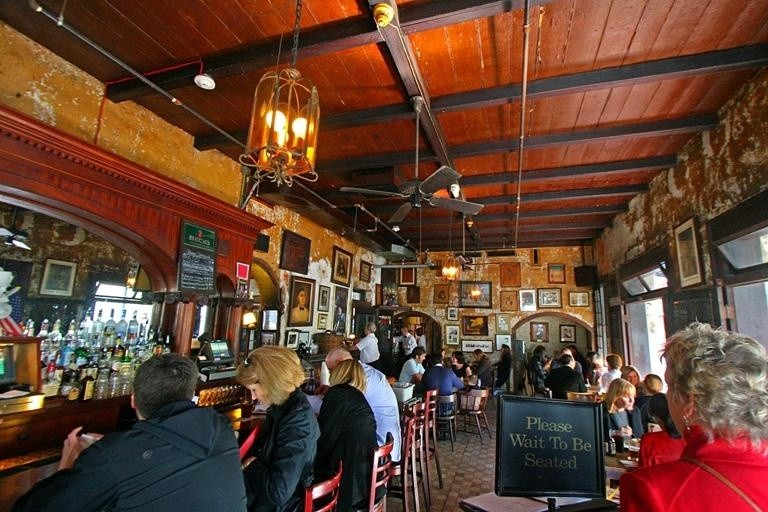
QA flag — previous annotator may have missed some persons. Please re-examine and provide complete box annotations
[417,328,426,352]
[314,359,388,511]
[334,307,346,331]
[619,321,768,511]
[399,343,512,437]
[12,351,248,511]
[291,289,308,322]
[399,327,417,356]
[325,346,401,465]
[235,344,323,511]
[526,345,685,470]
[350,322,381,369]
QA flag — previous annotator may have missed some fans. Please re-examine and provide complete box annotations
[340,95,484,226]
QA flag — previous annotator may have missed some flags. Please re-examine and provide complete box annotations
[0,290,28,336]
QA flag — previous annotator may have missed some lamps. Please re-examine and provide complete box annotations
[194,73,216,91]
[237,0,322,196]
[441,207,459,281]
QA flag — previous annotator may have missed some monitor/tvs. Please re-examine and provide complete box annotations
[210,340,233,358]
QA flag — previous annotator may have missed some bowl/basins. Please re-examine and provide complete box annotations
[565,390,599,403]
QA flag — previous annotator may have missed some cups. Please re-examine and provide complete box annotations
[602,428,640,461]
[93,366,133,394]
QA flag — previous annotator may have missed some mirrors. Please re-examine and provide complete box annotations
[0,187,168,348]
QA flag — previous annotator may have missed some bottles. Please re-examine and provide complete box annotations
[199,384,243,406]
[62,370,93,402]
[40,360,59,386]
[305,368,316,394]
[23,306,168,372]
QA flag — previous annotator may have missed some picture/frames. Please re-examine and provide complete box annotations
[672,216,705,289]
[258,226,422,356]
[433,248,590,353]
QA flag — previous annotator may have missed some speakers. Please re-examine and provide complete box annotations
[574,266,599,288]
[253,234,269,253]
[380,263,396,286]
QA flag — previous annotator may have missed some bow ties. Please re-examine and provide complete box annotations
[300,307,304,310]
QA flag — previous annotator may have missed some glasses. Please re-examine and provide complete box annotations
[243,358,254,369]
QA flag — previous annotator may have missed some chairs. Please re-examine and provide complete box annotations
[301,375,494,512]
